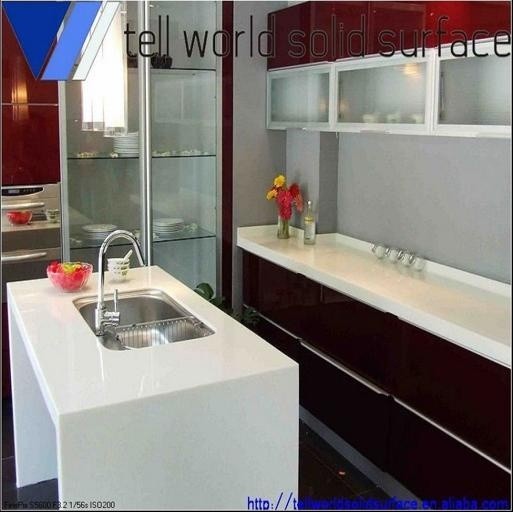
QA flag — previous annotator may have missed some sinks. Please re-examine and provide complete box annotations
[73,285,215,349]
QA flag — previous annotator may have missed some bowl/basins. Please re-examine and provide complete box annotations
[106,258,131,280]
[361,111,424,125]
[7,211,31,225]
[152,56,172,70]
[45,260,92,291]
[128,58,139,68]
[46,209,59,223]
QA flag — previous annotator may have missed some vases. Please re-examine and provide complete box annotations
[276,217,289,239]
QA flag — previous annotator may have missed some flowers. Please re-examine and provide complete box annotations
[266,174,304,220]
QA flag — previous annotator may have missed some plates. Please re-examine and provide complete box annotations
[113,132,139,157]
[81,223,118,242]
[152,218,186,238]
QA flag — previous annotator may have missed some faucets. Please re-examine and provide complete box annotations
[95,230,146,308]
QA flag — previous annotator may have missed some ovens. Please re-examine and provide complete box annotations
[0,184,66,307]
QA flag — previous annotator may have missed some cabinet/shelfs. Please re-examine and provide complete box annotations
[433,1,512,139]
[302,263,389,511]
[58,1,223,310]
[242,235,300,423]
[267,1,334,133]
[389,299,511,510]
[334,1,432,136]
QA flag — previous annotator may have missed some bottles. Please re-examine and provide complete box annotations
[303,200,316,245]
